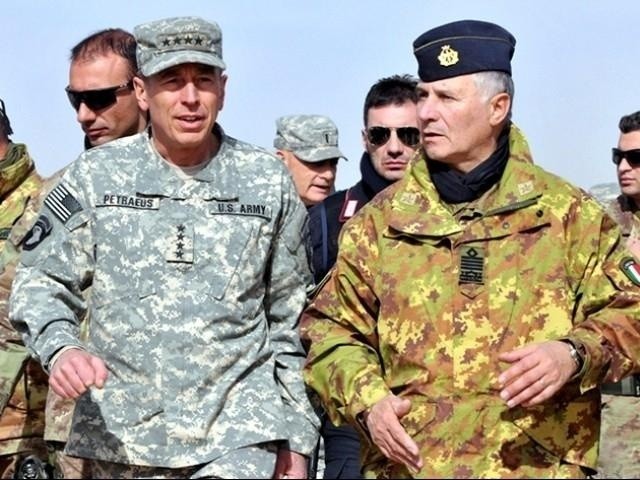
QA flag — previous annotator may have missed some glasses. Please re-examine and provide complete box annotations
[612,147,640,168]
[65,80,134,113]
[365,124,421,148]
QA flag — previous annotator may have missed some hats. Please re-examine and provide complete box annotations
[273,114,349,164]
[413,19,515,82]
[134,16,226,78]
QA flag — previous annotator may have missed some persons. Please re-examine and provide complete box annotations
[0,27,153,479]
[301,72,422,480]
[271,112,350,209]
[299,21,639,480]
[602,110,640,263]
[0,96,54,480]
[7,16,325,479]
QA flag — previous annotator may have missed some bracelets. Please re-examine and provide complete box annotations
[568,342,582,368]
